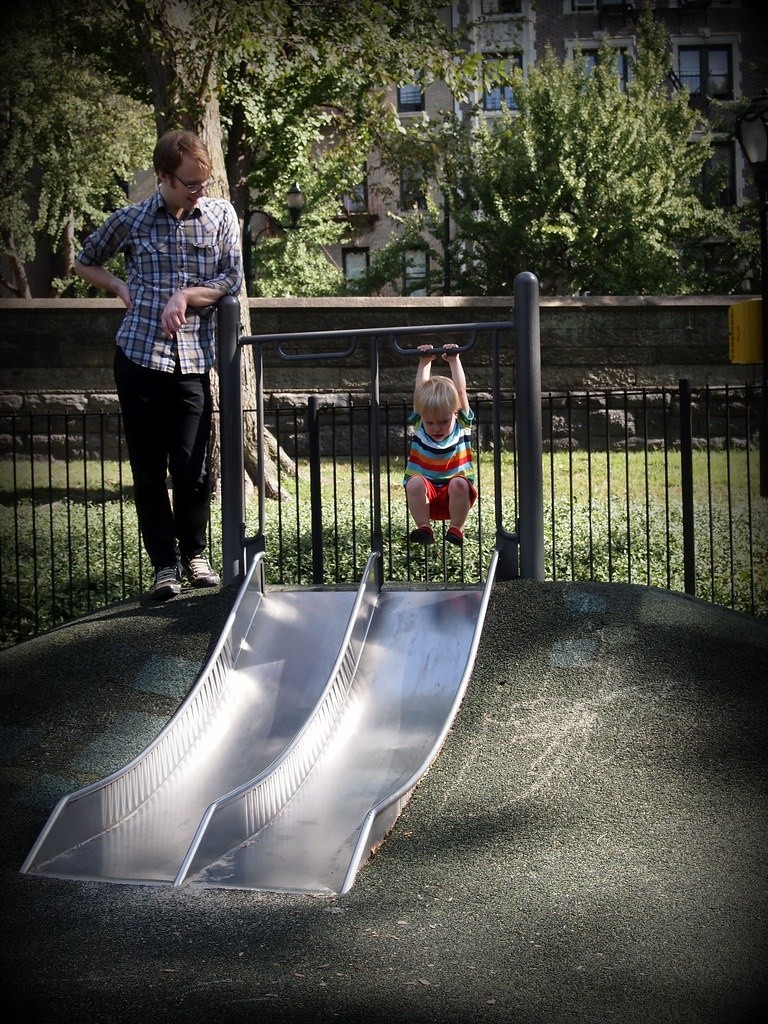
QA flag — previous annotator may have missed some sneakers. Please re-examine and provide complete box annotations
[149,564,181,597]
[180,555,221,587]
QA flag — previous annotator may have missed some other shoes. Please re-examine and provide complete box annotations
[410,526,434,545]
[445,527,464,547]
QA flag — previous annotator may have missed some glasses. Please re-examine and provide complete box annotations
[165,170,216,194]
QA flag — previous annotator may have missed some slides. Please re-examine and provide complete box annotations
[19,547,499,898]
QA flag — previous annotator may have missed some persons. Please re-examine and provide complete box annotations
[74,130,244,597]
[403,344,477,547]
